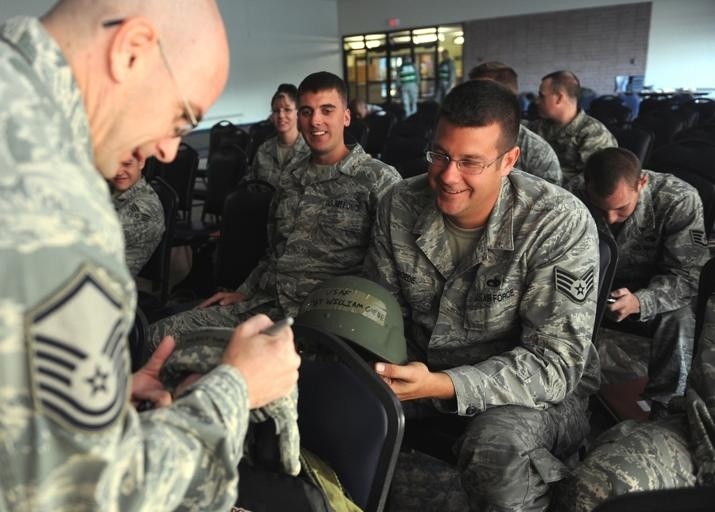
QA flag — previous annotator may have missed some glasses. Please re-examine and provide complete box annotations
[426,148,514,175]
[102,16,199,136]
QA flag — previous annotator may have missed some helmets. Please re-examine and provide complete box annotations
[158,324,304,477]
[292,275,408,368]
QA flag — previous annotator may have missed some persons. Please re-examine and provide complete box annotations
[110,70,406,364]
[473,60,713,512]
[2,2,300,512]
[396,54,418,120]
[354,78,600,512]
[438,48,455,104]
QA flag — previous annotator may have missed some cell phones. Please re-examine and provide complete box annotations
[607,296,616,304]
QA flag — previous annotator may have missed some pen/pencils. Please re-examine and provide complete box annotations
[261,318,294,336]
